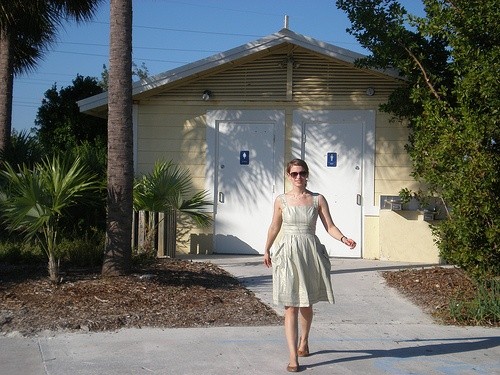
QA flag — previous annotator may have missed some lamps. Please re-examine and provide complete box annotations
[203,90,210,101]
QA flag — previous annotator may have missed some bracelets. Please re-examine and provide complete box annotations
[341,236,347,243]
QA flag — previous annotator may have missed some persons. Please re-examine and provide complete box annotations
[264,159,356,372]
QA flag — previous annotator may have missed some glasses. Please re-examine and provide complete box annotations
[289,170,307,178]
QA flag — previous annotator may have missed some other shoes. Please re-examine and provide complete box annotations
[298,335,310,357]
[287,362,299,372]
